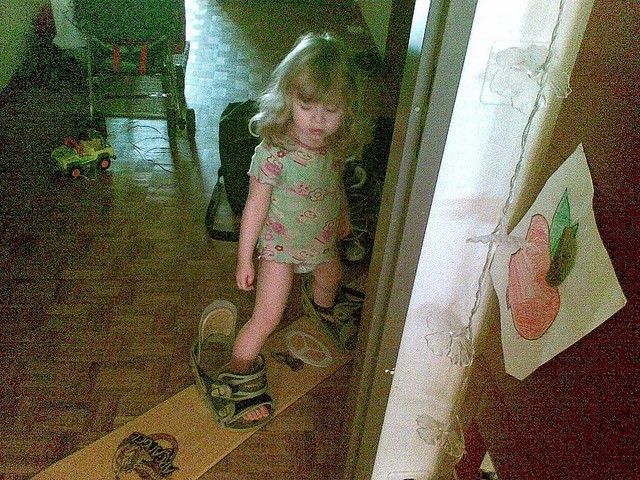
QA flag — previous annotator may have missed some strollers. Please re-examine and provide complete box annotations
[81,23,197,142]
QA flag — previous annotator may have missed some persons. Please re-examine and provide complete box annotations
[231,33,374,421]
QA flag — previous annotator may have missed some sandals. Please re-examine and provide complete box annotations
[302,277,365,356]
[191,302,275,431]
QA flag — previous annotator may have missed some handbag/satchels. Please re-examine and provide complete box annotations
[204,102,266,242]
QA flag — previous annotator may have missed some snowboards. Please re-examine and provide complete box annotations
[27,269,370,480]
